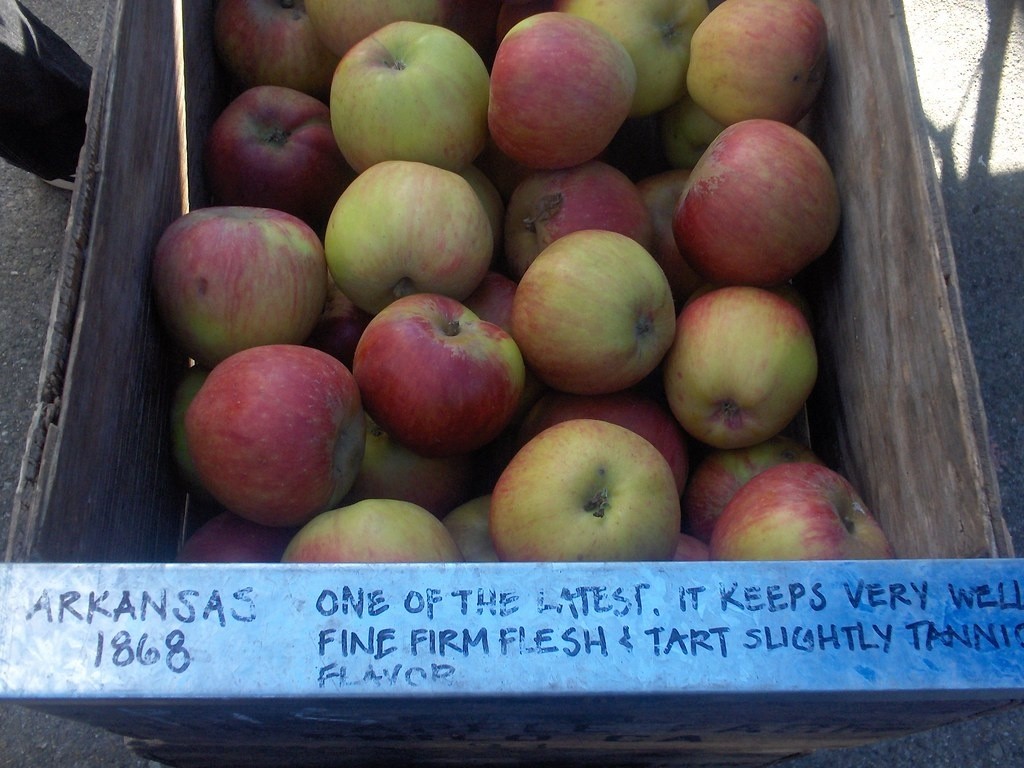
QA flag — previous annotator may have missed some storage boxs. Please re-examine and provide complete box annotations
[0,0,1024,768]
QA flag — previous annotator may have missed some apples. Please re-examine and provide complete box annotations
[149,0,896,563]
